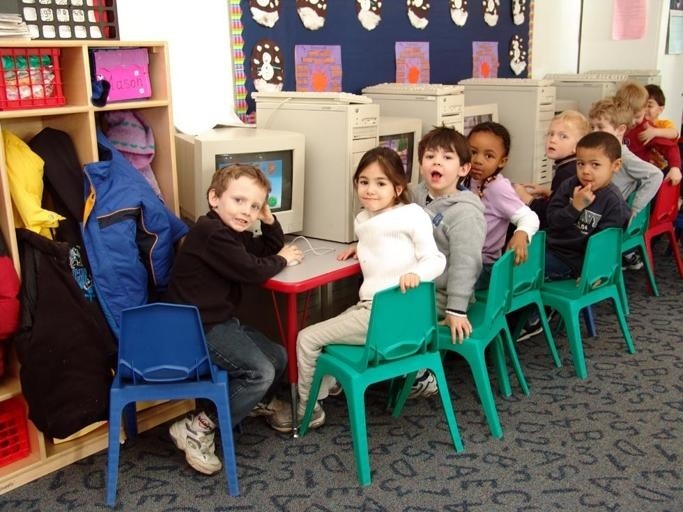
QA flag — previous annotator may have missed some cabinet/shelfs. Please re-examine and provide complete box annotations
[0,39,195,496]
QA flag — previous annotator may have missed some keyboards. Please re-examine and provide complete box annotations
[586,69,659,76]
[256,92,370,104]
[544,73,628,82]
[458,78,554,87]
[361,82,464,94]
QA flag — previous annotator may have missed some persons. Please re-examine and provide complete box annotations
[159,163,305,477]
[502,82,683,343]
[266,145,447,433]
[336,121,487,403]
[457,121,541,291]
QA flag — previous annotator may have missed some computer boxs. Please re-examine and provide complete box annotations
[554,83,616,118]
[629,76,660,87]
[363,94,465,141]
[458,87,556,186]
[256,104,380,243]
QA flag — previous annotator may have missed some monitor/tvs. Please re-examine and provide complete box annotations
[464,104,498,137]
[554,99,577,115]
[175,127,306,234]
[380,116,421,191]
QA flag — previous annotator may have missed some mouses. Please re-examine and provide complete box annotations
[287,253,304,266]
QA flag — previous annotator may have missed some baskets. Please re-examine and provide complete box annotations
[0,401,32,468]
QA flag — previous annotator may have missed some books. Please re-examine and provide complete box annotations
[0,13,31,41]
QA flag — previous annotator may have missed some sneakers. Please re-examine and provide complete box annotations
[408,371,438,400]
[247,400,327,433]
[622,252,643,272]
[515,314,542,343]
[169,417,223,476]
